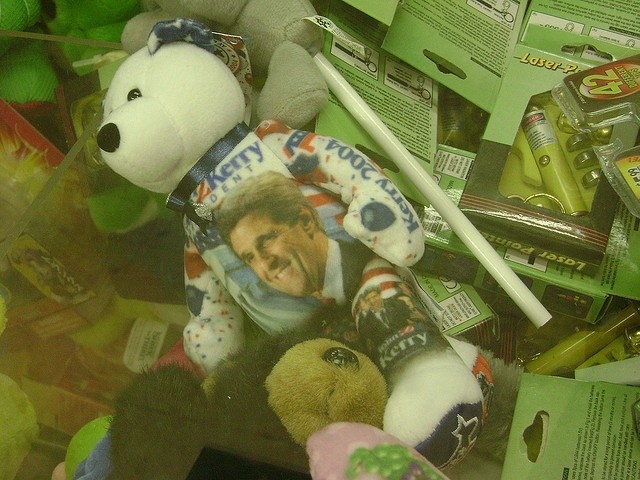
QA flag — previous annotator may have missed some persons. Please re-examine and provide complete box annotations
[213,170,373,316]
[357,284,407,342]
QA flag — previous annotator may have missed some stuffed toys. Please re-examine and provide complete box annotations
[53,304,450,478]
[96,19,491,471]
[121,0,330,131]
